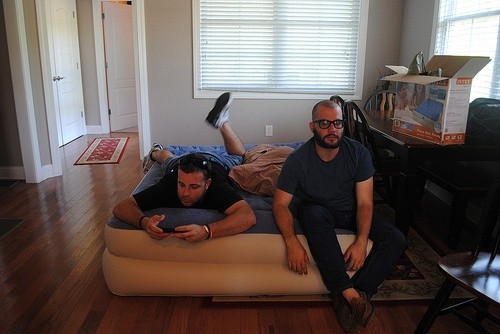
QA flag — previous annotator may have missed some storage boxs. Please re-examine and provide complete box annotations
[378,54,492,147]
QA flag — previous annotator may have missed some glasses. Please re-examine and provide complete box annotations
[314,119,345,129]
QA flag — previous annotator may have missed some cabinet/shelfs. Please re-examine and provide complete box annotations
[352,108,500,259]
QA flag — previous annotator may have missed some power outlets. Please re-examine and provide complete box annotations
[266,125,274,136]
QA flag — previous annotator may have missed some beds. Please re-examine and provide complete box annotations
[101,139,373,298]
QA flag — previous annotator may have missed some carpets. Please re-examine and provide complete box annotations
[72,136,129,166]
[200,188,477,308]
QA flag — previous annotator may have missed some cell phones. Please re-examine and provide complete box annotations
[162,228,187,233]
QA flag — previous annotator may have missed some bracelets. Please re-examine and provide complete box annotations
[139,215,150,229]
[202,223,213,240]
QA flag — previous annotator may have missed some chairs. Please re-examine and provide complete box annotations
[331,88,500,334]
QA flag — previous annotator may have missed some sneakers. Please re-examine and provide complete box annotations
[206,91,232,129]
[347,291,373,328]
[332,295,357,331]
[143,143,162,172]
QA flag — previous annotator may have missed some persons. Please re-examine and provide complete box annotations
[112,91,257,242]
[407,83,425,111]
[272,99,407,332]
[396,82,409,111]
